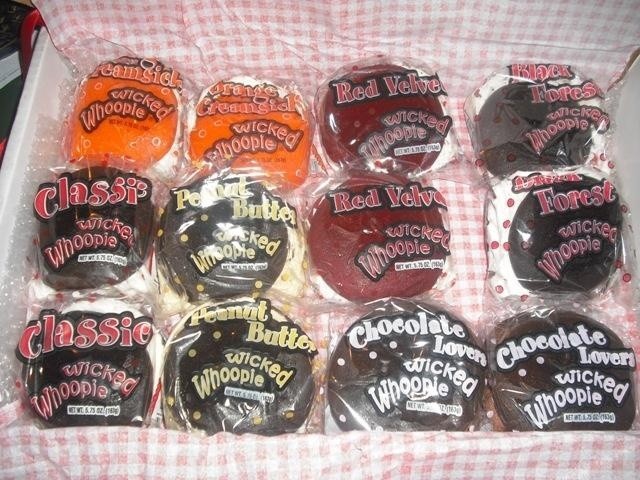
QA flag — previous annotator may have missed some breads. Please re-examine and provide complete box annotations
[320,297,488,431]
[182,72,317,196]
[302,170,453,305]
[9,297,167,426]
[461,61,615,180]
[29,164,155,300]
[480,306,637,432]
[482,166,627,309]
[312,52,462,176]
[155,173,304,305]
[63,50,195,171]
[161,294,322,436]
